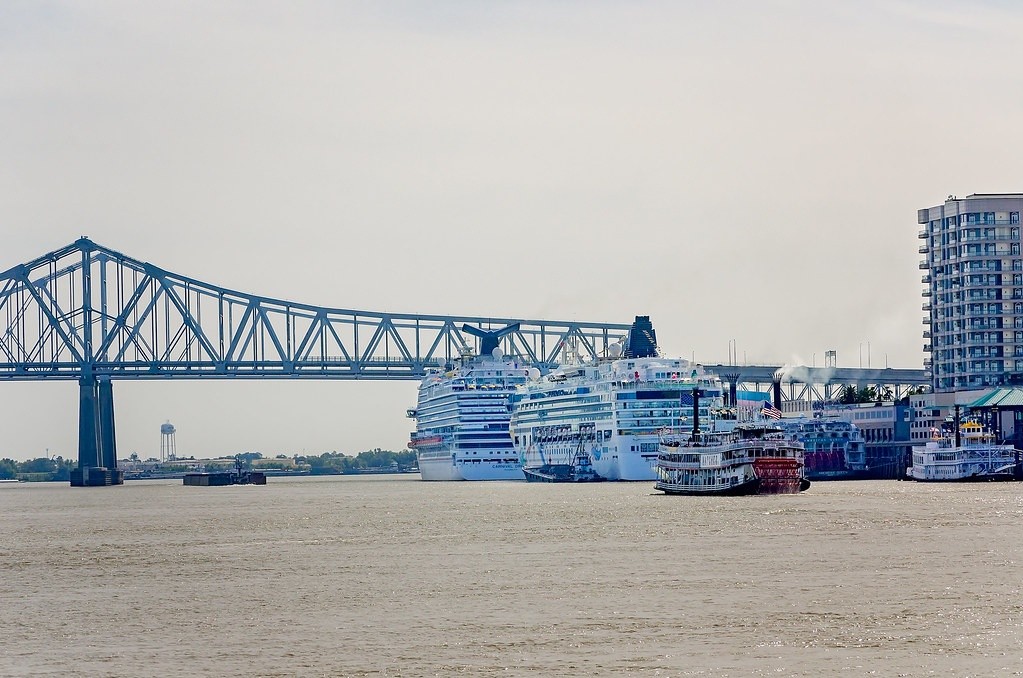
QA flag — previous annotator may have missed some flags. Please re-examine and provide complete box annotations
[764,401,780,420]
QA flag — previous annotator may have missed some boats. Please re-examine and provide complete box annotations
[520,433,608,483]
[407,436,443,449]
[724,372,870,481]
[653,387,811,497]
[534,426,595,441]
[905,403,1023,483]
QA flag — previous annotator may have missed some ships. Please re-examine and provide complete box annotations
[508,316,723,481]
[407,322,540,481]
